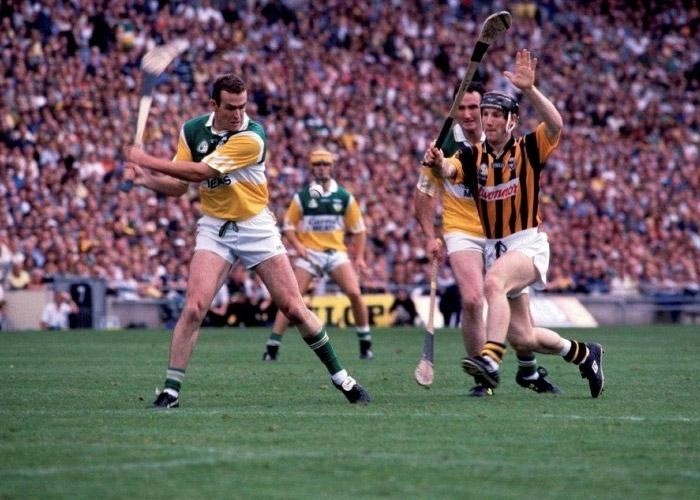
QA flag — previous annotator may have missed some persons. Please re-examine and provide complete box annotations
[405,80,564,400]
[122,73,377,411]
[1,0,700,335]
[259,147,377,365]
[418,46,607,403]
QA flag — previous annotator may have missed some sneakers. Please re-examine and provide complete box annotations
[579,342,604,397]
[462,356,500,397]
[262,352,278,361]
[146,392,179,408]
[331,348,372,404]
[515,366,563,394]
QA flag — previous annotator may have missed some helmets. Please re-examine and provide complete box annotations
[309,150,333,172]
[480,92,520,113]
